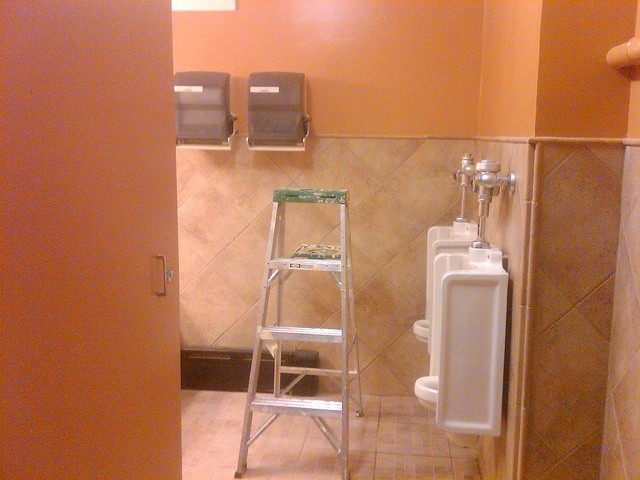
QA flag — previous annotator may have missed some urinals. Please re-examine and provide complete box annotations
[413,220,478,355]
[414,246,509,448]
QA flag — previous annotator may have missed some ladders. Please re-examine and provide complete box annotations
[234,191,362,480]
